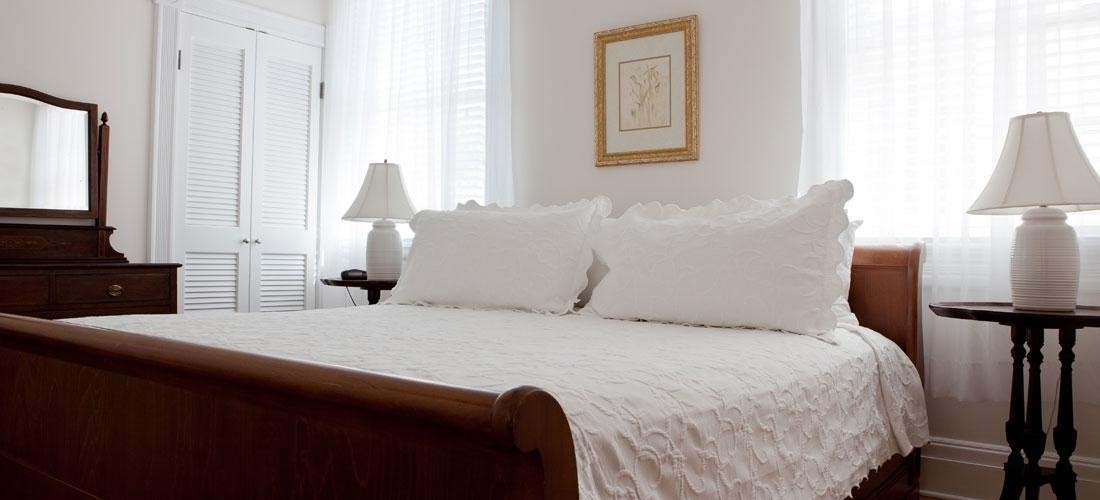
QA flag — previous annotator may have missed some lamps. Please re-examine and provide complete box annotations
[341,160,415,282]
[967,113,1099,309]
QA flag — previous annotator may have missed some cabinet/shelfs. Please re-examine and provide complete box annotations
[155,10,325,312]
[5,260,180,318]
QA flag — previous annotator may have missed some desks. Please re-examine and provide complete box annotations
[929,299,1100,499]
[323,276,397,303]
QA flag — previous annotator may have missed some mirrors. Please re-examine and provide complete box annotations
[0,84,100,220]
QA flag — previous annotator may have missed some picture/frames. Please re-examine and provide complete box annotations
[593,14,701,167]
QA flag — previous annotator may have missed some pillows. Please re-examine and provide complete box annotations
[386,195,615,316]
[578,180,854,346]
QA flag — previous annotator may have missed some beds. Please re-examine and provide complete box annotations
[1,245,928,499]
